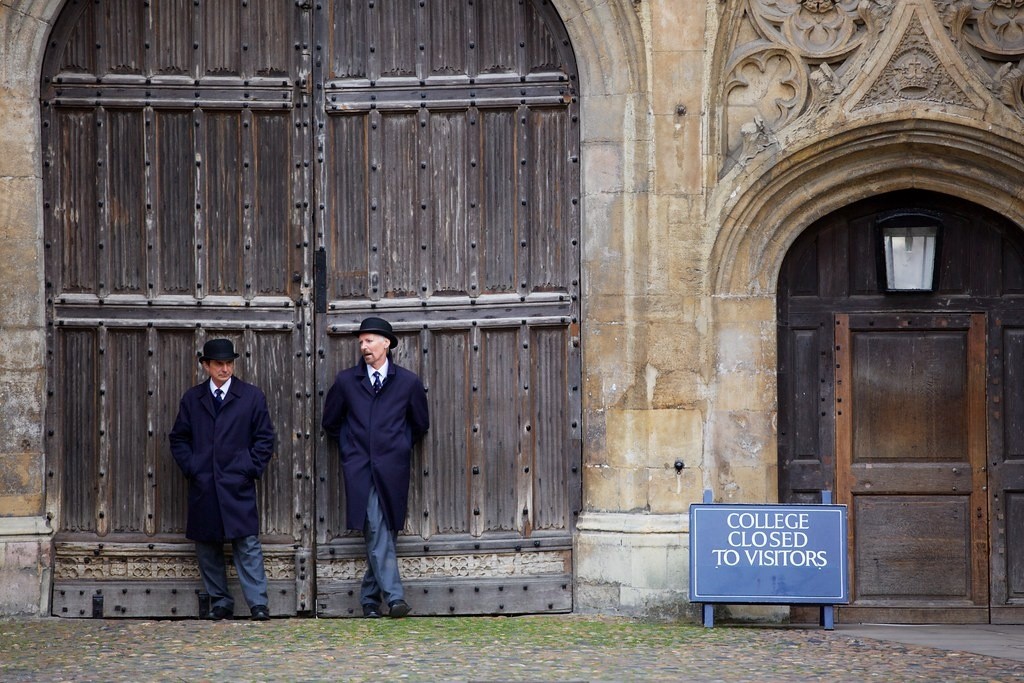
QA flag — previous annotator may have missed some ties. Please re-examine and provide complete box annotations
[215,389,222,413]
[373,371,382,395]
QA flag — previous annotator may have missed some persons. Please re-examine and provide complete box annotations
[168,339,275,620]
[319,317,431,617]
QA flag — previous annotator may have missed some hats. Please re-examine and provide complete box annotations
[352,317,399,350]
[198,338,240,363]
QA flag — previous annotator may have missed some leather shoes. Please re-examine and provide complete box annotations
[204,606,234,620]
[389,599,412,617]
[363,603,381,618]
[252,605,270,620]
